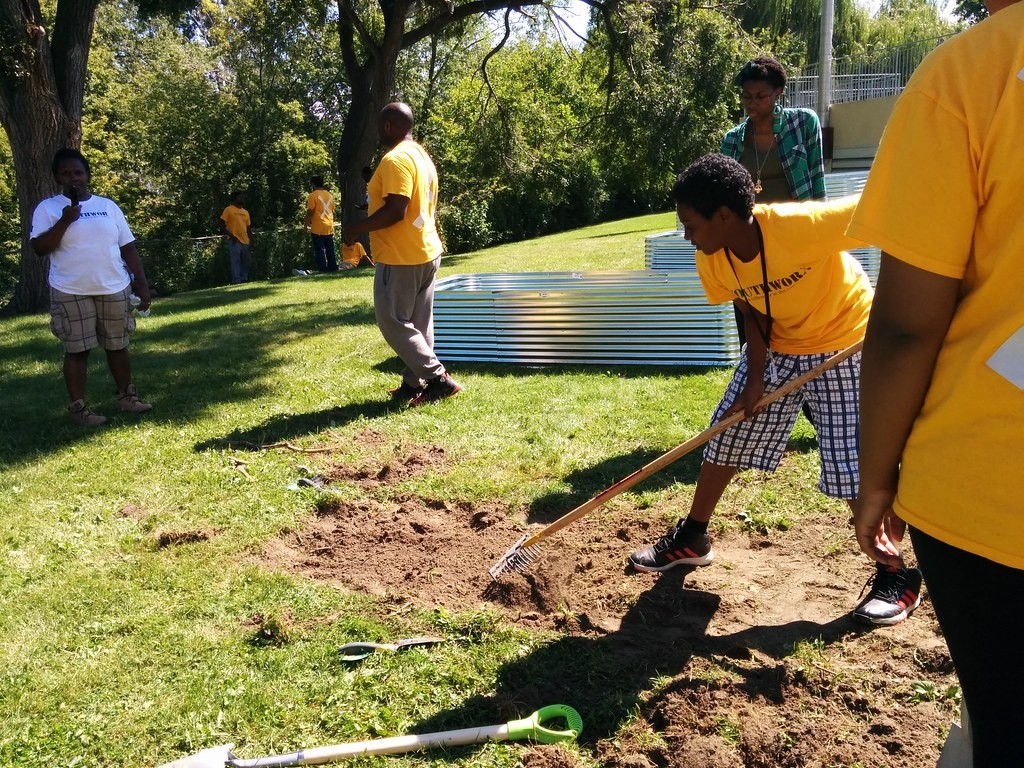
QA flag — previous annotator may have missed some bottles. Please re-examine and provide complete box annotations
[130,294,150,316]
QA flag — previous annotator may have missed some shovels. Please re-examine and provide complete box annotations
[151,700,586,768]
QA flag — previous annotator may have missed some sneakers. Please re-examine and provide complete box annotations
[388,382,423,397]
[853,564,922,625]
[410,373,460,407]
[628,518,713,571]
[68,400,107,426]
[116,384,152,413]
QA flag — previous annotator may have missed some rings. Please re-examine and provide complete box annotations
[147,301,151,305]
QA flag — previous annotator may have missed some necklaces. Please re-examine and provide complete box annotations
[751,125,776,194]
[730,248,758,259]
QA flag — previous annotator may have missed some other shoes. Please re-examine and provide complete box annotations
[292,269,305,276]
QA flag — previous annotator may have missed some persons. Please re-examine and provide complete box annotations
[844,0,1024,768]
[341,101,462,407]
[30,150,153,426]
[219,189,253,284]
[629,152,924,626]
[302,174,337,273]
[718,58,827,205]
[337,232,374,272]
